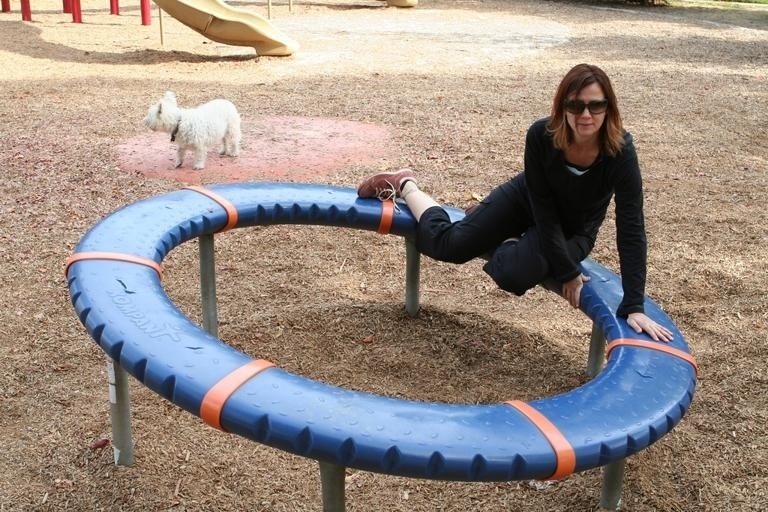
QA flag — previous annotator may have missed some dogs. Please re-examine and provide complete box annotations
[142,91,241,170]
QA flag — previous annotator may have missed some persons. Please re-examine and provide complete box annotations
[356,63,674,344]
